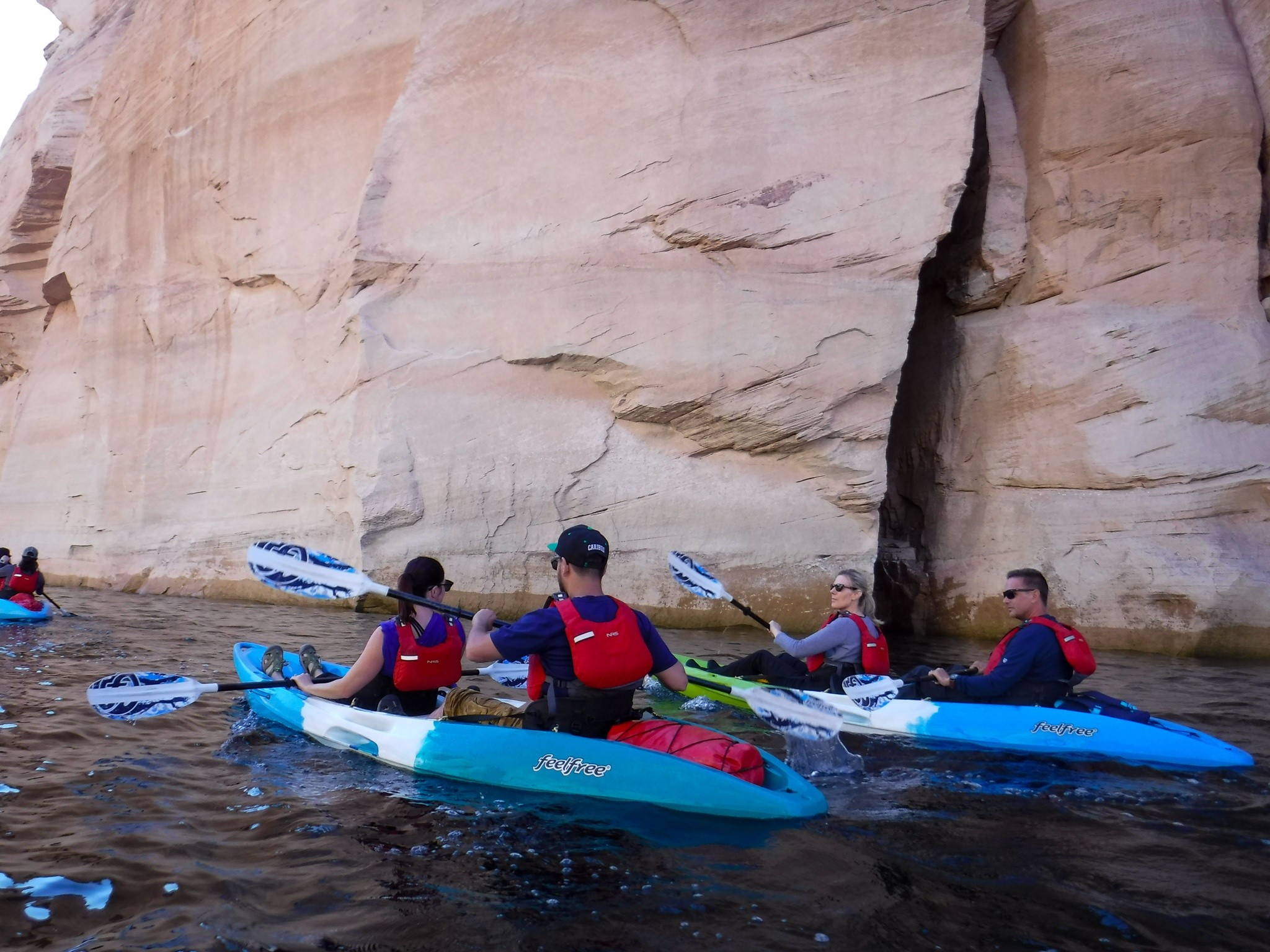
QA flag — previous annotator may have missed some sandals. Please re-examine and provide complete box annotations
[298,644,324,678]
[261,645,285,677]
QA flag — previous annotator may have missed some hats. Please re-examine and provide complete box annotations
[22,546,38,558]
[547,524,610,570]
[0,547,12,558]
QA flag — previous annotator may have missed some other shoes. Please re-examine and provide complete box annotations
[377,694,408,716]
[685,659,704,670]
[707,659,725,669]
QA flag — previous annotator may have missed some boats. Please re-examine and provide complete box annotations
[0,591,55,620]
[654,651,1255,774]
[231,640,828,823]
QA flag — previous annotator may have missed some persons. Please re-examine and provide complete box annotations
[378,524,687,738]
[830,567,1072,705]
[0,547,44,599]
[685,568,880,693]
[262,555,465,716]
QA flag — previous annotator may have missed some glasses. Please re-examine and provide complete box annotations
[830,583,857,592]
[427,579,454,592]
[1002,588,1042,599]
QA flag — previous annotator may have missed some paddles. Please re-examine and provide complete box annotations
[7,560,78,617]
[87,651,530,720]
[247,538,845,741]
[841,667,979,712]
[667,550,771,631]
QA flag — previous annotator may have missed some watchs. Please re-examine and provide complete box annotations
[949,674,958,690]
